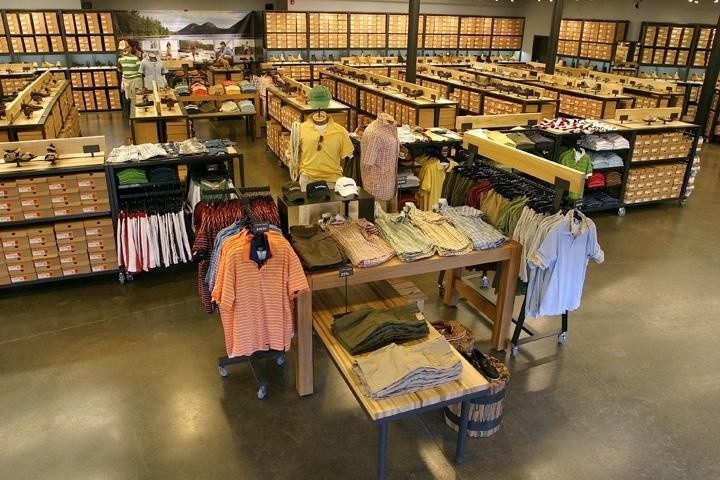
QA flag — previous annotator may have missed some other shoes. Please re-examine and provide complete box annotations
[418,54,517,64]
[326,66,392,86]
[398,85,424,97]
[417,65,452,80]
[270,70,308,101]
[548,59,679,97]
[460,64,545,99]
[270,52,406,65]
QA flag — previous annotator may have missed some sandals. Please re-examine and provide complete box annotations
[2,142,60,163]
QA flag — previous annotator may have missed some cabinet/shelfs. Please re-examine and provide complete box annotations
[0,11,122,292]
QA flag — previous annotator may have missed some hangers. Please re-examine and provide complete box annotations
[237,207,267,247]
[196,186,269,207]
[122,194,186,219]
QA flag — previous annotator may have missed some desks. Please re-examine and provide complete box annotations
[310,296,492,479]
[288,205,524,396]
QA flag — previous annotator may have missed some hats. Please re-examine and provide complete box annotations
[282,176,361,203]
[117,39,130,51]
[306,83,333,111]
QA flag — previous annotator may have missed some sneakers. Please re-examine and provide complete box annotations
[0,60,64,124]
[459,347,502,383]
[133,86,177,110]
[642,113,673,124]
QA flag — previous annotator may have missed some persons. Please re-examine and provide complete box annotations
[191,46,196,58]
[285,84,356,192]
[117,40,143,101]
[241,40,251,54]
[138,53,167,90]
[216,41,236,68]
[359,110,400,204]
[165,41,173,61]
